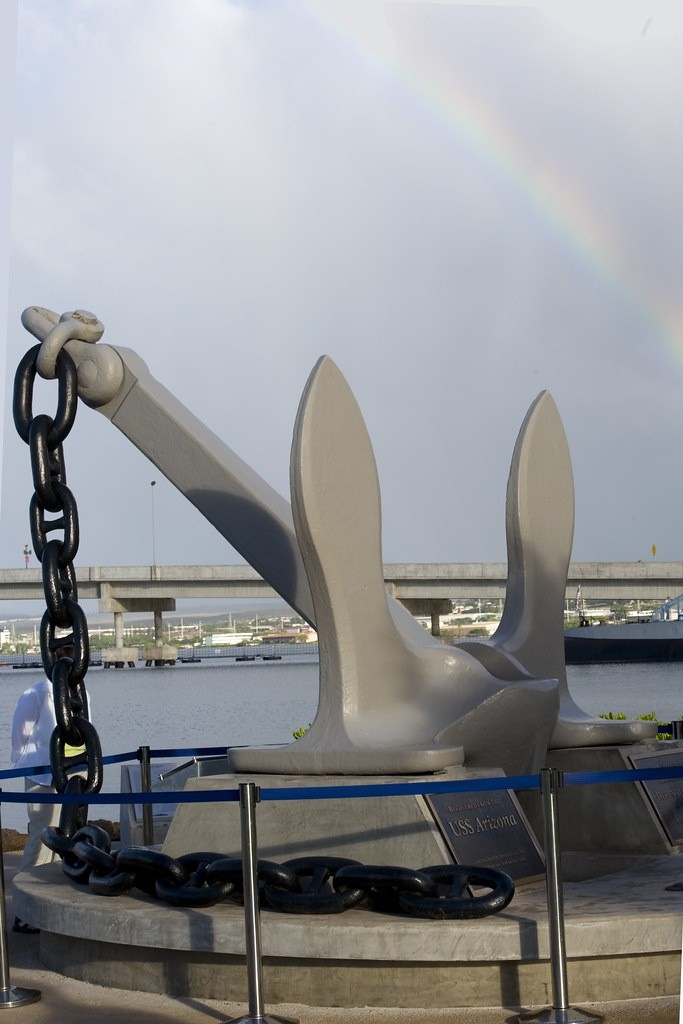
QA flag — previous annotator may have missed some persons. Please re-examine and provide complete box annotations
[664,595,673,620]
[11,677,91,935]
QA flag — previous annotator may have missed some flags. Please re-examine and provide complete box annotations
[575,585,581,611]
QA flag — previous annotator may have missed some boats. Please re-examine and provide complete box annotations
[564,594,680,665]
